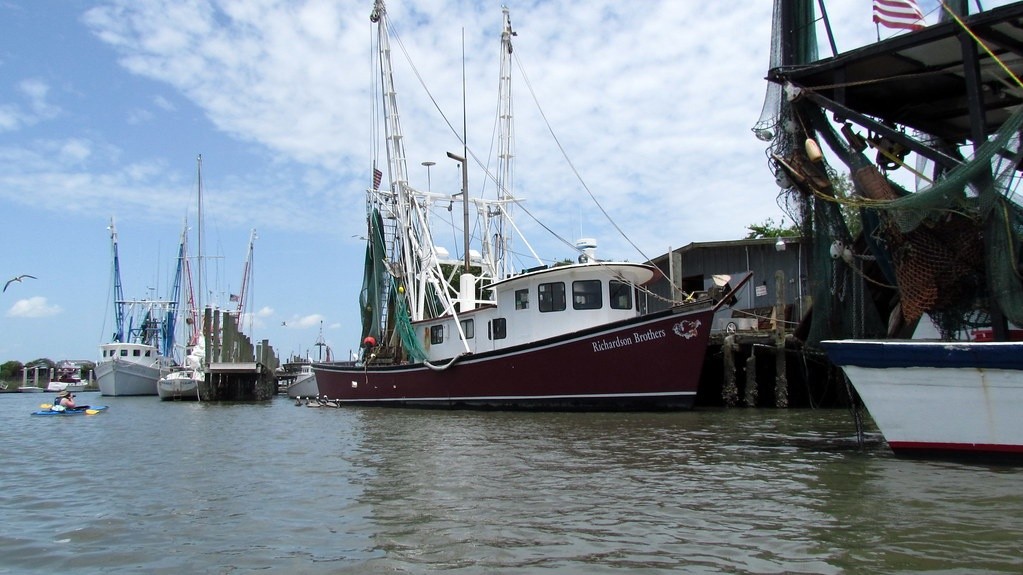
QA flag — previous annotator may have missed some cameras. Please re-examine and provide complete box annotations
[67,393,77,397]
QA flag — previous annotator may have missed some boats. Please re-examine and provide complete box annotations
[286,358,320,400]
[43,382,88,393]
[30,403,108,416]
[817,334,1023,463]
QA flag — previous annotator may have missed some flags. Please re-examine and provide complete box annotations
[873,0,928,31]
[230,294,240,302]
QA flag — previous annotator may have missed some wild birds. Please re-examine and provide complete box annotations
[3,274,38,294]
[294,394,341,408]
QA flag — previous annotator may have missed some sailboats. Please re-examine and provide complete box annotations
[91,153,258,400]
[307,0,755,414]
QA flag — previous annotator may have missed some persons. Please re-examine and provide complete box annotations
[54,391,75,409]
